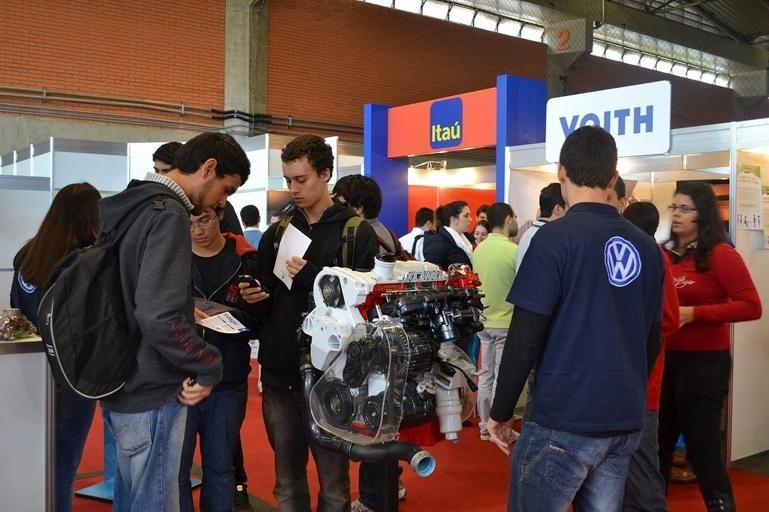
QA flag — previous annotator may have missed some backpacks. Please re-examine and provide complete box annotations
[37,195,183,400]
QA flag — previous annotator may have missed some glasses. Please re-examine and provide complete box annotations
[190,214,219,232]
[667,204,695,212]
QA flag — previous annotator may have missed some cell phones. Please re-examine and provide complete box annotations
[239,273,259,289]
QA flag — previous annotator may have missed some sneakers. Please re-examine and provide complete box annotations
[350,500,372,512]
[481,428,522,443]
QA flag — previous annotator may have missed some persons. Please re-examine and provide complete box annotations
[241,133,406,511]
[8,179,106,512]
[396,173,679,511]
[488,125,664,511]
[95,133,224,511]
[656,178,764,511]
